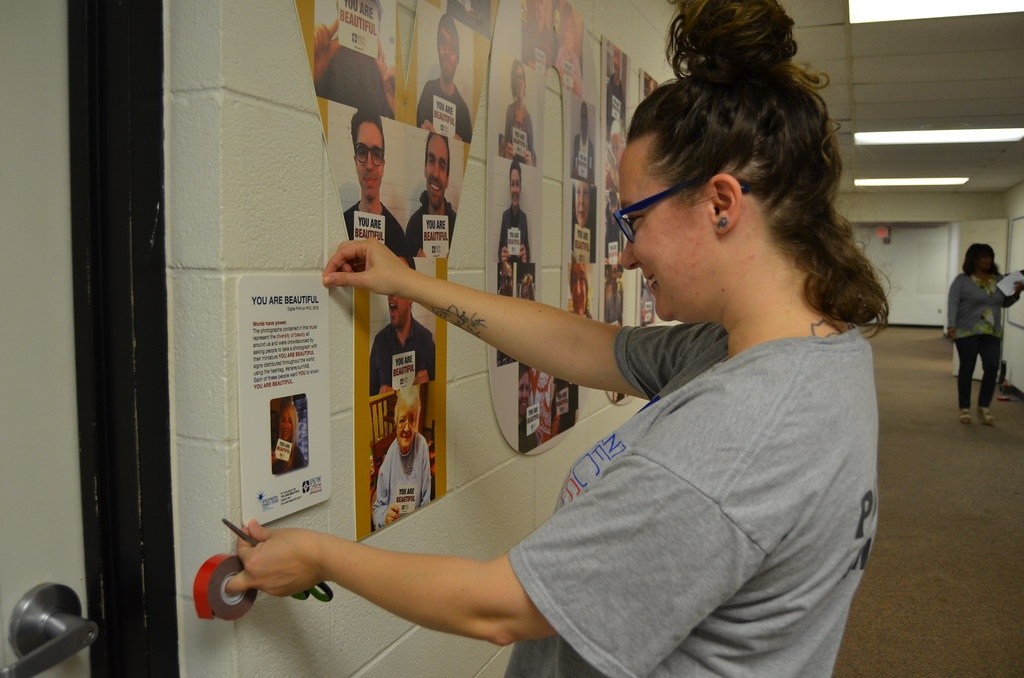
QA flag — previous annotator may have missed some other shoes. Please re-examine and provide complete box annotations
[978,406,995,425]
[960,408,970,425]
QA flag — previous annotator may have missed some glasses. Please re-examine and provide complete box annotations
[614,172,750,245]
[397,418,417,428]
[355,142,385,166]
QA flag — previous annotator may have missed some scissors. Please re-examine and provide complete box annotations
[221,517,334,603]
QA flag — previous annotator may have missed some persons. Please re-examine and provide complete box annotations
[604,265,623,325]
[571,100,596,184]
[405,132,458,255]
[341,109,416,269]
[605,46,628,189]
[494,162,534,263]
[518,363,580,455]
[944,242,1024,426]
[496,264,536,366]
[567,261,593,321]
[224,2,889,678]
[372,387,433,530]
[271,405,308,474]
[603,189,624,270]
[499,59,536,166]
[417,13,473,145]
[314,0,396,116]
[639,275,656,325]
[571,181,597,263]
[369,257,448,395]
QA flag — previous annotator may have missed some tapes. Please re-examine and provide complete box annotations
[191,552,257,621]
